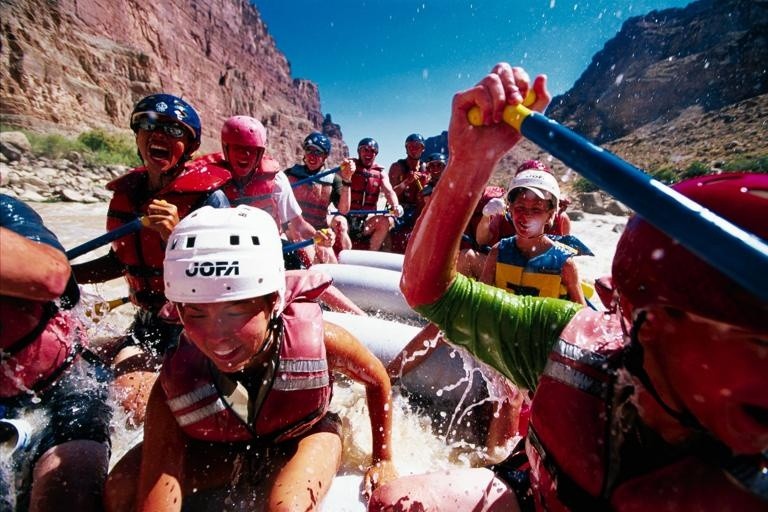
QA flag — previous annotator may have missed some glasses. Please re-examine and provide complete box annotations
[361,148,375,154]
[136,116,185,139]
[304,148,322,156]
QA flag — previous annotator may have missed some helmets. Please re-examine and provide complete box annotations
[223,115,266,166]
[515,161,555,175]
[130,93,201,154]
[405,134,425,147]
[509,169,559,214]
[303,132,330,156]
[613,173,768,336]
[358,138,378,154]
[426,153,446,172]
[164,205,286,317]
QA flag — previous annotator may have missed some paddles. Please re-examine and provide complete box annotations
[88,228,329,327]
[501,209,594,301]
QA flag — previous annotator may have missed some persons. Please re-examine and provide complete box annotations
[476,168,588,458]
[457,158,571,283]
[219,115,336,269]
[401,58,768,512]
[69,93,231,428]
[0,193,113,512]
[329,138,404,251]
[103,204,393,512]
[416,153,446,208]
[284,132,356,266]
[382,134,431,252]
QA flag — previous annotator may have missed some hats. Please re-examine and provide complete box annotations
[508,186,551,201]
[305,144,324,153]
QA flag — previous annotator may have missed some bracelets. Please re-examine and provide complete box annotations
[342,179,352,186]
